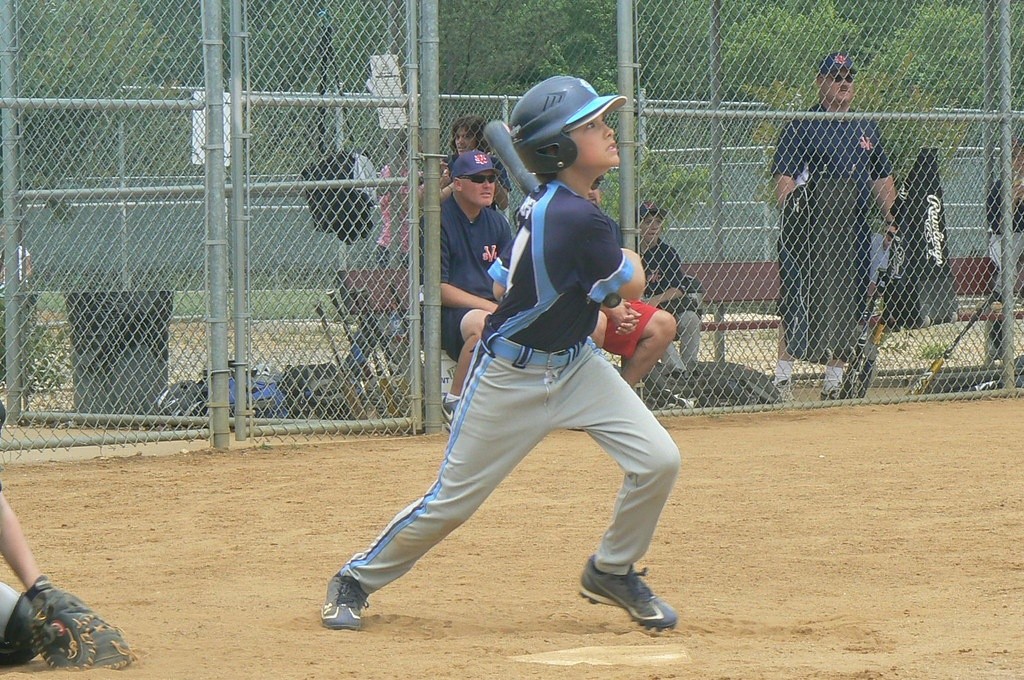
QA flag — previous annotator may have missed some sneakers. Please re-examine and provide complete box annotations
[577,555,678,633]
[321,572,368,629]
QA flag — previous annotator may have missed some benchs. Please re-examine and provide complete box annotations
[338,259,1024,365]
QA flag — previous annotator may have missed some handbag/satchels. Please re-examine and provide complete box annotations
[303,150,374,242]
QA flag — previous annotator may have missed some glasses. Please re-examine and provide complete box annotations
[591,181,600,190]
[458,174,496,183]
[820,74,854,83]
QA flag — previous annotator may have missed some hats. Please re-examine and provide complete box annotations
[817,51,857,75]
[635,198,667,222]
[452,149,494,179]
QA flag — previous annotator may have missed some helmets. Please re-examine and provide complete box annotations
[509,75,628,173]
[157,380,208,428]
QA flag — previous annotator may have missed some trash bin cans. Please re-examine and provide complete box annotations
[0,291,39,385]
[61,289,176,426]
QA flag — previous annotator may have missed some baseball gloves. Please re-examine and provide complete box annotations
[27,588,138,670]
[152,362,346,419]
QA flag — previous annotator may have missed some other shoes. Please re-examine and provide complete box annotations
[441,394,459,432]
[820,382,843,400]
[776,379,796,402]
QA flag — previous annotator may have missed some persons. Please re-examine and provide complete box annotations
[345,52,1024,435]
[0,398,137,672]
[320,76,681,629]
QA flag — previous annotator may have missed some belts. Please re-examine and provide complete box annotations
[490,334,587,369]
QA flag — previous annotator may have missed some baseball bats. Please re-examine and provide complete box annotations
[483,120,622,308]
[906,289,1000,395]
[311,283,426,421]
[839,268,902,400]
